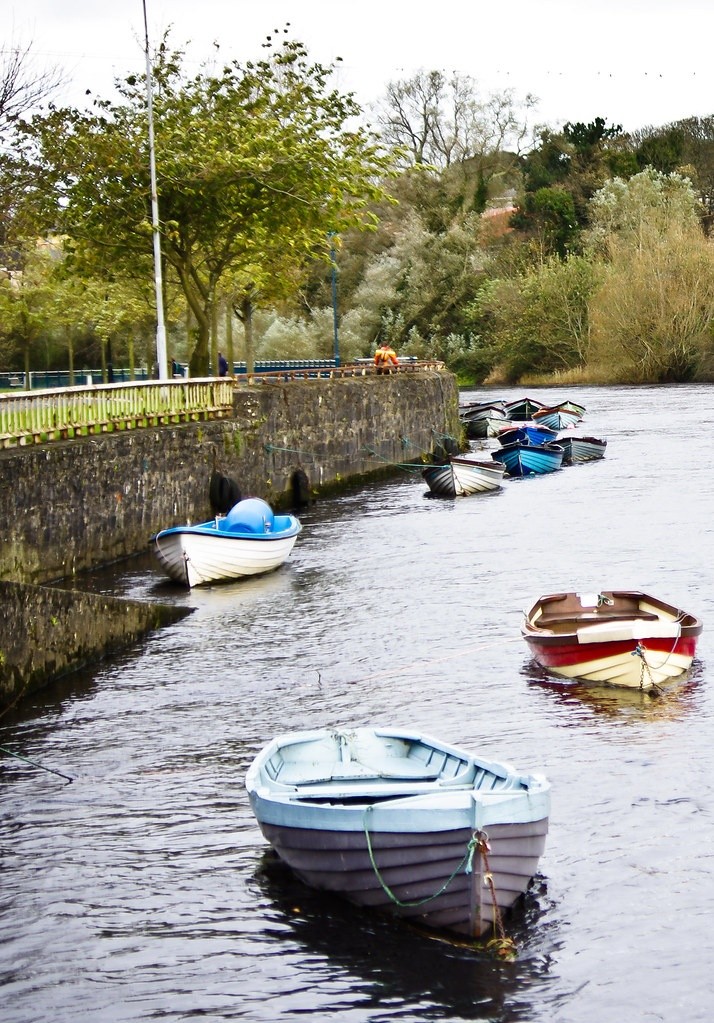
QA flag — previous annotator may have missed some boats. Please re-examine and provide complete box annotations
[459,398,607,476]
[245,727,551,936]
[146,496,303,588]
[421,453,506,496]
[520,589,704,691]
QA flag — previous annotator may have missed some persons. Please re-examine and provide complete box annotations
[374,341,400,375]
[170,358,179,377]
[218,351,228,377]
